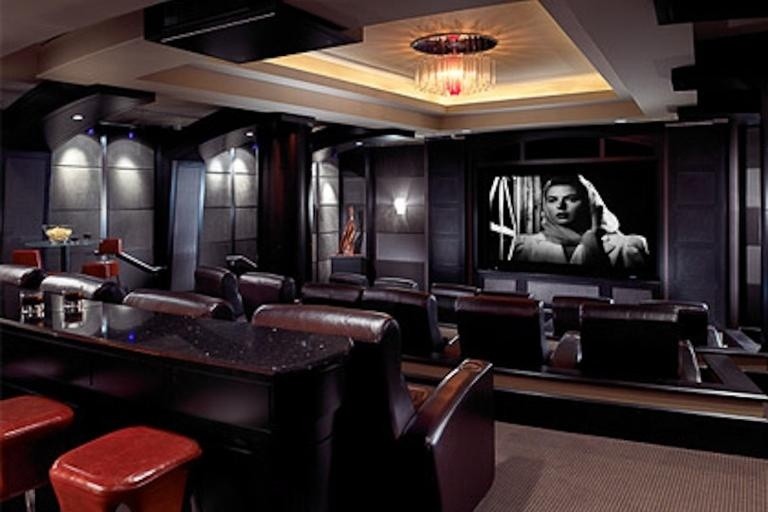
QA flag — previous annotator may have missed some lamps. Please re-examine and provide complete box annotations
[393,197,407,215]
[410,32,498,97]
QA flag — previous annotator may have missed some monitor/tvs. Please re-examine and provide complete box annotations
[472,157,664,292]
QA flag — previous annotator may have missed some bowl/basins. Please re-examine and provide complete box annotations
[41,225,73,244]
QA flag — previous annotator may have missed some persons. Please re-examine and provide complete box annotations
[507,173,650,265]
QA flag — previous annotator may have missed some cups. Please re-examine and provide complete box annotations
[69,230,92,243]
[62,306,86,328]
[63,284,85,309]
[19,287,46,314]
